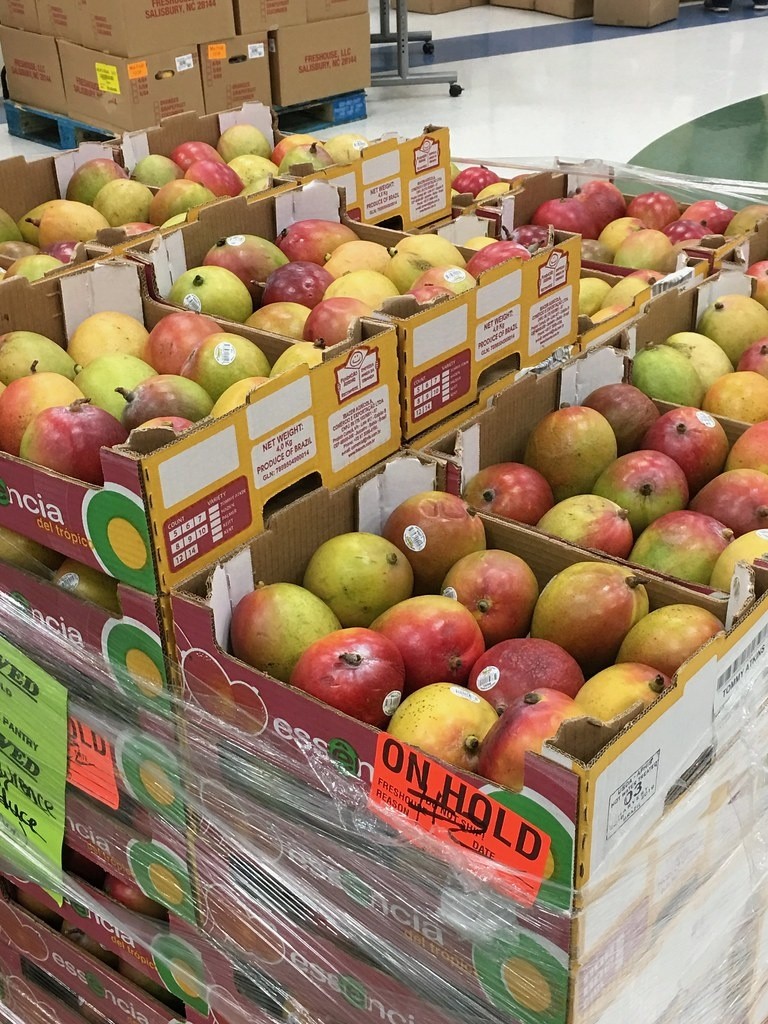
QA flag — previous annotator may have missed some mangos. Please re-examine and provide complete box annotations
[1,123,768,1003]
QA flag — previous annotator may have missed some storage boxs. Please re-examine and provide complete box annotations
[0,0,768,1021]
